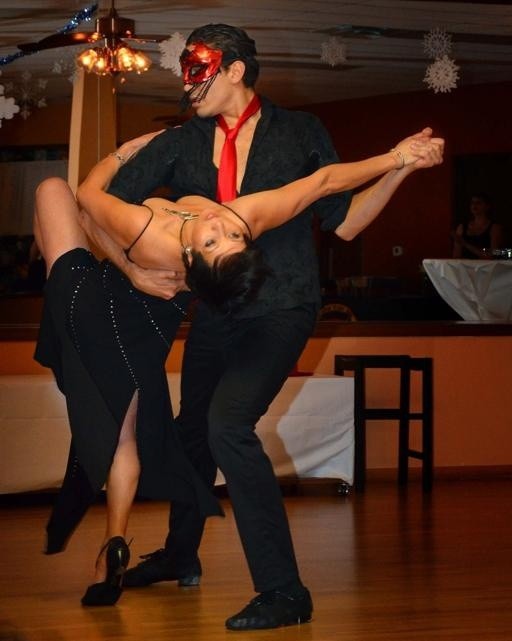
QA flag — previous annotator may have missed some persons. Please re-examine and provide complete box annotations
[77,24,444,632]
[450,193,503,262]
[32,128,445,606]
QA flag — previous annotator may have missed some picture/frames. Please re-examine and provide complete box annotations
[333,349,437,495]
[1,371,361,500]
[409,247,509,323]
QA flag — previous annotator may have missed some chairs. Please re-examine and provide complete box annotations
[122,547,204,589]
[225,584,316,631]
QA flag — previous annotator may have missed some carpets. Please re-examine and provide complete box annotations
[213,93,261,201]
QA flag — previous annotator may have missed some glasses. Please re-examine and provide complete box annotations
[68,4,154,78]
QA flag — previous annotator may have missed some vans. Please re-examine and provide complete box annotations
[79,535,131,607]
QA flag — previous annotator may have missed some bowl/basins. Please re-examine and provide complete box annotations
[166,209,198,251]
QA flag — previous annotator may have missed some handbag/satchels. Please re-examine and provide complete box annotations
[110,150,127,165]
[389,148,405,170]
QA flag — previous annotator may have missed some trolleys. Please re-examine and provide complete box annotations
[15,0,172,53]
